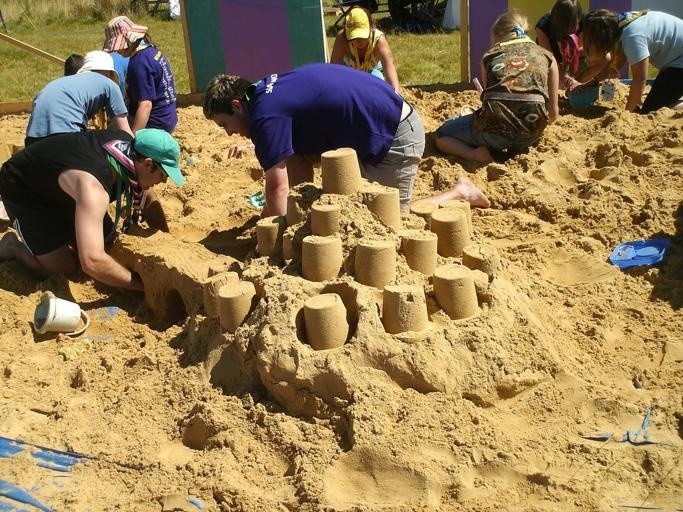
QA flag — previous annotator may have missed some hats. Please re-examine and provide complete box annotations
[77,51,119,85]
[345,7,373,41]
[102,15,149,52]
[134,127,185,187]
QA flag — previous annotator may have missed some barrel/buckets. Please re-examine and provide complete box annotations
[33,291,83,335]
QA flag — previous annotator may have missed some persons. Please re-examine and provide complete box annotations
[578,9,683,117]
[531,0,612,100]
[61,51,130,117]
[0,126,188,295]
[23,50,135,150]
[202,62,493,225]
[100,15,178,235]
[328,4,402,100]
[432,7,563,170]
